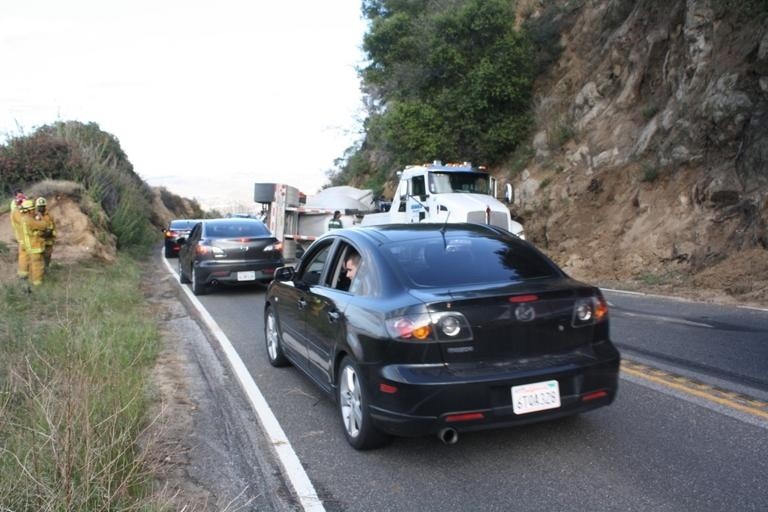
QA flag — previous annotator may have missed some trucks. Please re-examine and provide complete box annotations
[252,161,526,261]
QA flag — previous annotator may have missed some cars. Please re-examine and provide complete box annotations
[261,210,621,452]
[161,211,285,295]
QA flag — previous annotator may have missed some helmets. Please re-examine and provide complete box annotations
[16,198,22,206]
[20,200,35,213]
[36,197,47,207]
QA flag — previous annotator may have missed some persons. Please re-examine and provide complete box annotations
[344,250,361,281]
[327,209,342,233]
[10,187,56,288]
